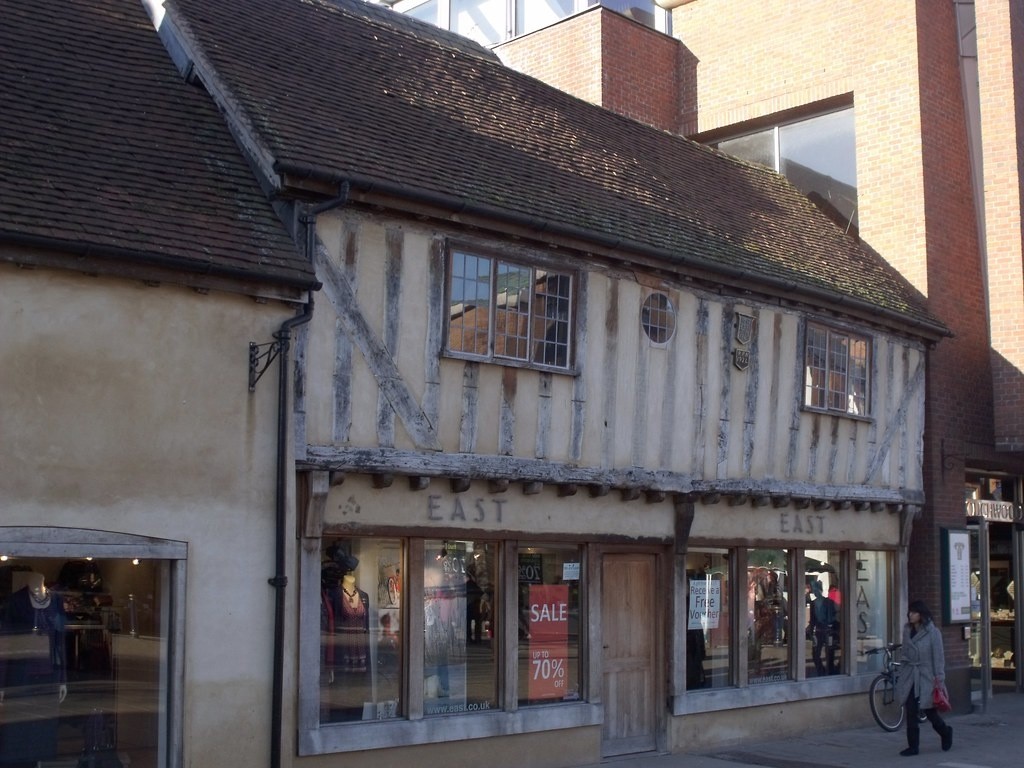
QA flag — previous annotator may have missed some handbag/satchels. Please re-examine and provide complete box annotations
[933,677,951,712]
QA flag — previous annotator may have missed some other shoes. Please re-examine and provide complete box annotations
[900,746,919,756]
[941,726,953,751]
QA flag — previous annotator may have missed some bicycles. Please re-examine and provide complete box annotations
[862,642,930,733]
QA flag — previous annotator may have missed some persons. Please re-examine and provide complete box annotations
[466,568,490,644]
[809,580,841,675]
[0,573,68,768]
[894,599,953,756]
[319,574,373,724]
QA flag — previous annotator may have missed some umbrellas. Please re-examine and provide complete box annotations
[706,546,835,577]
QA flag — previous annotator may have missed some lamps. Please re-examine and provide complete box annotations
[434,539,448,561]
[322,541,360,572]
